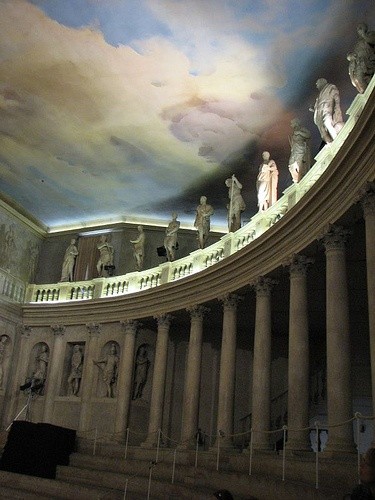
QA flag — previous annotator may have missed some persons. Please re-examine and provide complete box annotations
[346,21,375,95]
[194,195,214,250]
[225,176,246,234]
[163,212,180,262]
[129,224,145,272]
[0,334,154,402]
[343,445,375,500]
[210,488,234,500]
[287,117,313,184]
[96,234,115,278]
[194,428,204,450]
[0,221,42,285]
[307,78,345,145]
[256,150,280,213]
[58,239,78,282]
